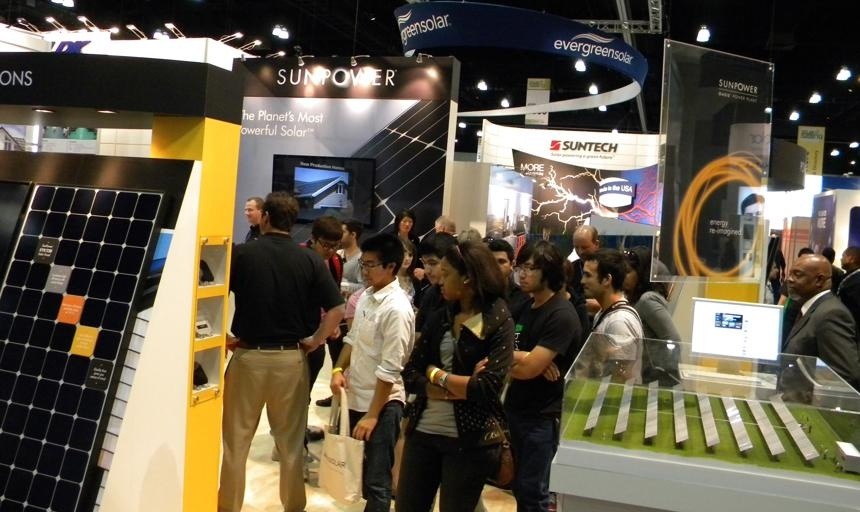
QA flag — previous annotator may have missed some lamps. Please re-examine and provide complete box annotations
[15,14,290,62]
[295,50,434,70]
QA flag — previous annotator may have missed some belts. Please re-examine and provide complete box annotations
[237,342,300,350]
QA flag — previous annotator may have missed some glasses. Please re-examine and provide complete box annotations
[313,238,343,251]
[357,257,384,272]
[510,263,543,277]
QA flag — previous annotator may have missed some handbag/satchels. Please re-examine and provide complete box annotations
[318,424,366,505]
[485,440,514,486]
[642,364,680,388]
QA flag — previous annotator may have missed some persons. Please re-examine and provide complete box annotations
[767,245,860,403]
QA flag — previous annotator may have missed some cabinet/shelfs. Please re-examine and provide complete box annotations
[188,236,233,407]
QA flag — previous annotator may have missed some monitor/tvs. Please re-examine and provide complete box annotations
[271,153,377,228]
[688,297,785,369]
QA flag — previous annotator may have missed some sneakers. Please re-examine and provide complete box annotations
[307,423,325,441]
[317,395,333,407]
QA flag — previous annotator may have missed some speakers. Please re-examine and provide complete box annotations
[768,138,806,192]
